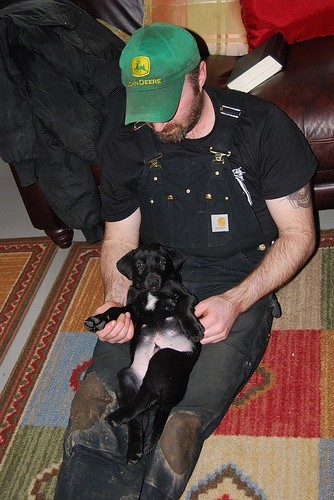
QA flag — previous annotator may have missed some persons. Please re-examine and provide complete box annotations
[51,23,318,498]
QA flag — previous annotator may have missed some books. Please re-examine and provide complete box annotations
[240,38,289,94]
[225,31,283,92]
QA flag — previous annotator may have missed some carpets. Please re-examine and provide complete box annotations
[0,229,334,500]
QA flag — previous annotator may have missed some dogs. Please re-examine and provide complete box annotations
[83,244,206,466]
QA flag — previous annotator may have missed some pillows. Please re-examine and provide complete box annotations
[240,0,334,53]
[142,0,248,56]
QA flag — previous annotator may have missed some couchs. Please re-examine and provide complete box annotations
[205,33,334,210]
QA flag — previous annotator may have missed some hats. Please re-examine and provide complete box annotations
[119,22,201,125]
